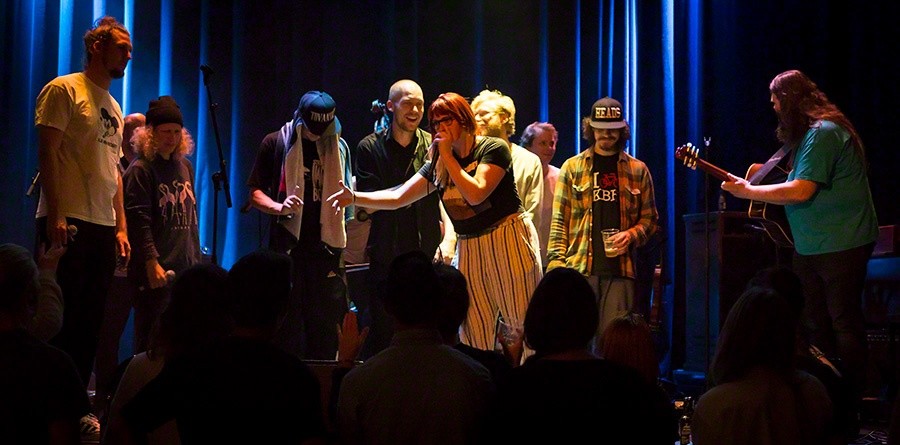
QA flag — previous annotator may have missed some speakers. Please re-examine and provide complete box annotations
[683,210,781,373]
[862,254,900,430]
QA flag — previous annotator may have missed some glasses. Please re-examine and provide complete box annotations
[431,116,454,130]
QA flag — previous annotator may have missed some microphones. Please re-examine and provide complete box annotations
[429,143,439,175]
[140,269,176,292]
[44,225,77,253]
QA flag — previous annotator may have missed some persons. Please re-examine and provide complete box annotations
[34,17,201,436]
[0,242,838,445]
[246,80,660,366]
[719,70,881,400]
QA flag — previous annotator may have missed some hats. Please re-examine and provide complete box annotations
[298,91,335,136]
[145,96,184,127]
[589,98,626,129]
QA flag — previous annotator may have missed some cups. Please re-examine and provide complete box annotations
[496,317,524,355]
[601,229,618,257]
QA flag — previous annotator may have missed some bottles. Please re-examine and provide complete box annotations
[679,396,694,445]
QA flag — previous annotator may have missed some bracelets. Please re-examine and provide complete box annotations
[354,193,356,204]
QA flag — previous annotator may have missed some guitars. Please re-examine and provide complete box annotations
[676,142,795,248]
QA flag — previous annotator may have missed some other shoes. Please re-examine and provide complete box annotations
[79,413,100,443]
